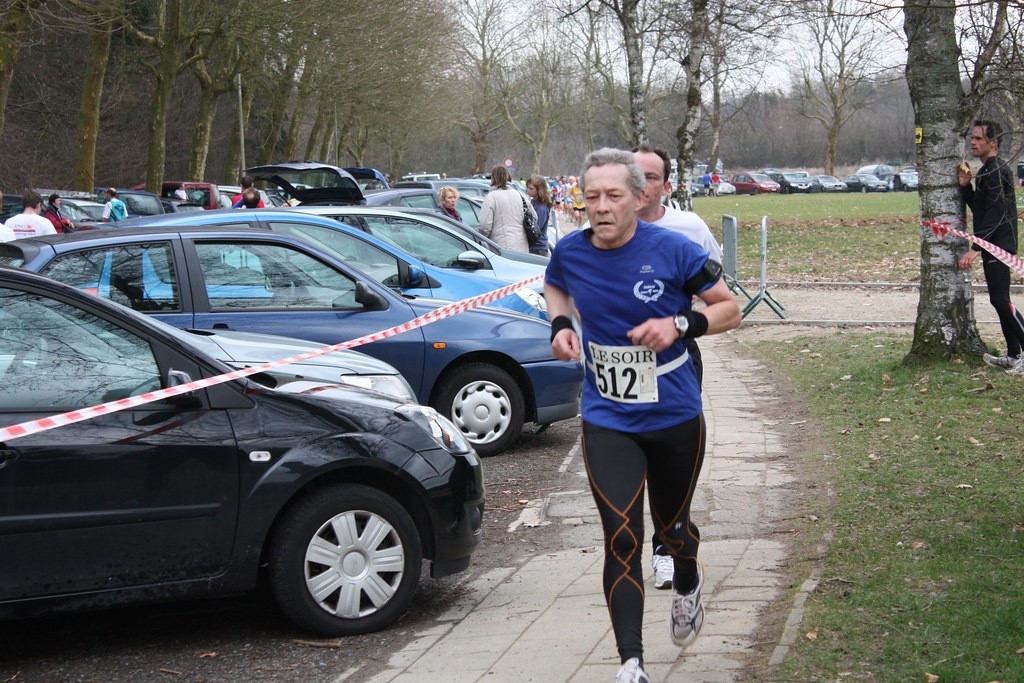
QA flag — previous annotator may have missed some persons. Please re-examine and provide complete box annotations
[544,148,741,683]
[701,171,712,197]
[547,175,586,230]
[436,186,464,224]
[711,172,720,197]
[959,119,1024,375]
[44,194,74,234]
[570,148,724,590]
[479,165,538,254]
[4,189,57,239]
[519,178,527,185]
[526,175,552,257]
[0,190,16,243]
[175,176,333,209]
[102,187,128,222]
[661,185,681,210]
[365,173,394,189]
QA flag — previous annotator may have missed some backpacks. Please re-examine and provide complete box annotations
[108,199,126,222]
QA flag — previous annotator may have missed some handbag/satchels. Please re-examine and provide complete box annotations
[518,190,542,245]
[62,225,73,233]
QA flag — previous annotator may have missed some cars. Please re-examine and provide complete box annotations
[809,174,849,193]
[1,328,418,406]
[727,171,781,195]
[761,168,814,194]
[892,166,919,192]
[856,165,895,181]
[845,173,890,194]
[0,221,586,459]
[1,263,488,641]
[668,163,737,196]
[1,160,551,297]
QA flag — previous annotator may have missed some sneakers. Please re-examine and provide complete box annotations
[1005,351,1024,376]
[983,352,1018,370]
[615,657,651,683]
[653,554,674,589]
[670,561,705,647]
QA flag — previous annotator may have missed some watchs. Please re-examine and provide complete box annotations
[674,313,689,339]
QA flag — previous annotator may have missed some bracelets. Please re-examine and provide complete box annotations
[678,309,709,339]
[550,315,576,344]
